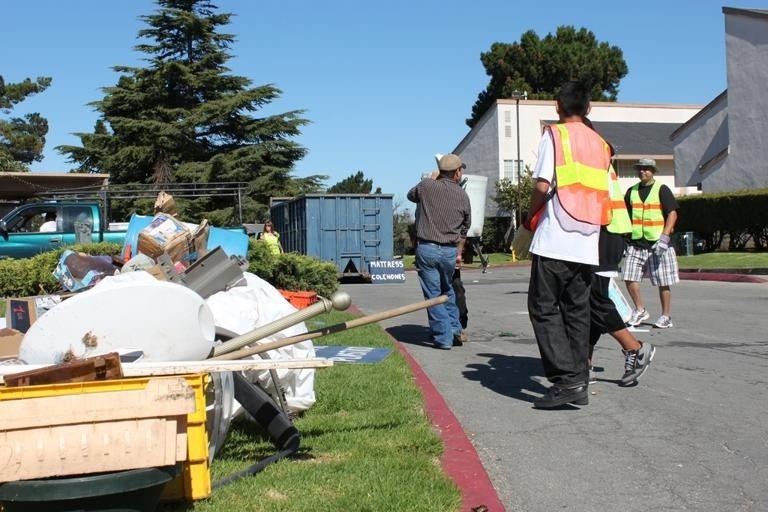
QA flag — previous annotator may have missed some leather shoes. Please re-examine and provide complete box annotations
[534,386,588,409]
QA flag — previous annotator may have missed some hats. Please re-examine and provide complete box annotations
[439,154,466,171]
[632,157,661,172]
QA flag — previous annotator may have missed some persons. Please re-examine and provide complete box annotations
[586,117,658,386]
[406,153,471,350]
[510,86,611,407]
[621,155,680,328]
[452,267,469,343]
[39,212,59,233]
[259,219,284,254]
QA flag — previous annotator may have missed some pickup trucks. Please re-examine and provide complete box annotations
[0,201,251,257]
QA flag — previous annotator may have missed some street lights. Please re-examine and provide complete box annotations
[512,89,530,228]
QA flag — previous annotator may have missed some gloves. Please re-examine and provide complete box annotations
[512,223,534,260]
[652,233,670,256]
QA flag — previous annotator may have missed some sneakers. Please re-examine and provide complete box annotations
[620,340,656,387]
[588,366,596,384]
[453,334,463,346]
[461,329,469,342]
[653,316,673,329]
[628,308,650,326]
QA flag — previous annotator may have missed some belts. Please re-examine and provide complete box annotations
[419,240,438,244]
